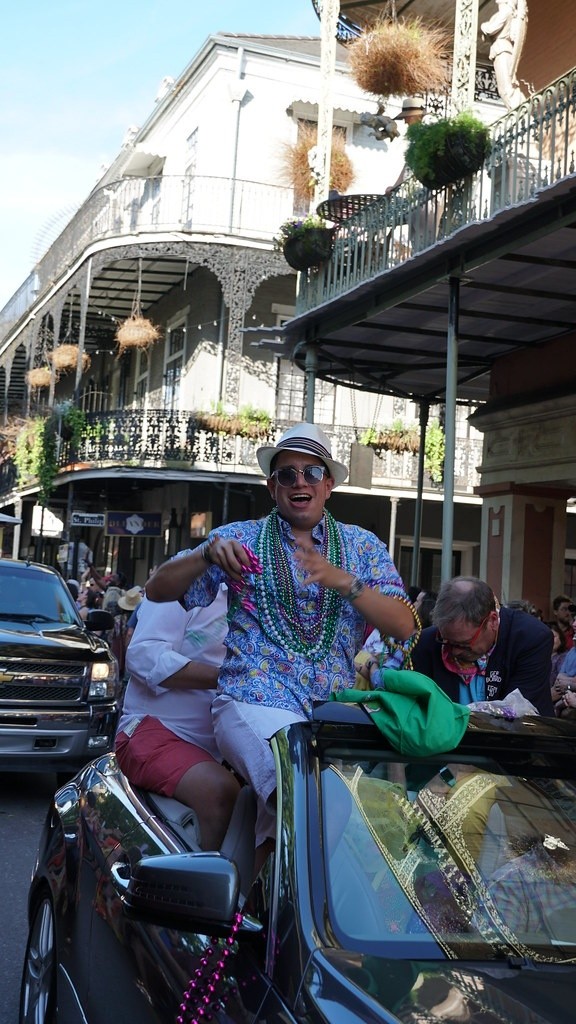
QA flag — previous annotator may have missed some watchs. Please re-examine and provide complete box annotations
[367,661,380,669]
[440,767,456,787]
[568,680,571,690]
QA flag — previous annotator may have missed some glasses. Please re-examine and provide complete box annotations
[436,616,489,651]
[271,466,326,488]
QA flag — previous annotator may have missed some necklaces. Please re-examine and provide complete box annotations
[453,642,496,684]
[227,542,263,609]
[254,502,350,661]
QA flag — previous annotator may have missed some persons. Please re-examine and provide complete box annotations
[355,573,576,938]
[142,424,422,874]
[52,546,159,680]
[114,546,242,852]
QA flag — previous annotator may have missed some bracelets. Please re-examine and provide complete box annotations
[87,563,96,567]
[345,577,365,599]
[358,664,362,672]
[200,535,219,562]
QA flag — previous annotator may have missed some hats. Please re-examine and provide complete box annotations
[118,589,143,610]
[256,424,348,489]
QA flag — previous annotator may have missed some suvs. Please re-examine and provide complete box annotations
[0,555,121,787]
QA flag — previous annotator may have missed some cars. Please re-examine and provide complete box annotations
[18,699,576,1024]
